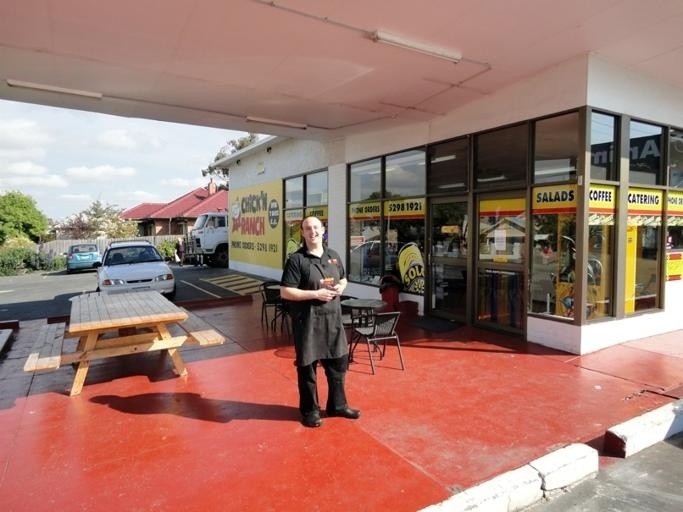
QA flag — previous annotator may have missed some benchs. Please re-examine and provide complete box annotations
[22,322,65,372]
[173,307,225,345]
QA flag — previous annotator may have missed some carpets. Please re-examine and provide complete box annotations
[411,316,465,332]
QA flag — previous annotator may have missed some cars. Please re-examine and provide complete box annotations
[347,237,404,279]
[62,243,103,273]
[95,240,176,297]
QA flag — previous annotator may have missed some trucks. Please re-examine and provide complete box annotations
[191,211,228,268]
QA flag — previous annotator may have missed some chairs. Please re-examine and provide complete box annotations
[258,279,404,374]
[111,253,122,262]
[139,250,149,256]
[635,273,655,310]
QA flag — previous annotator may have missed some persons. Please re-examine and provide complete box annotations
[278,215,363,428]
[173,238,186,267]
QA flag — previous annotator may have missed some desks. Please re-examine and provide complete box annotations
[68,289,187,395]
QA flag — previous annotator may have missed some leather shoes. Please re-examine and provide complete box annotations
[299,408,321,427]
[325,407,361,418]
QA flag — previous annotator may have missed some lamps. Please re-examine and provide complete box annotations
[245,115,307,131]
[370,30,462,64]
[5,79,102,100]
